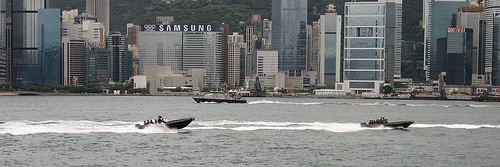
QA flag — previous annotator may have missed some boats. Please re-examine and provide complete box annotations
[361,117,414,129]
[134,115,195,129]
[192,95,247,103]
